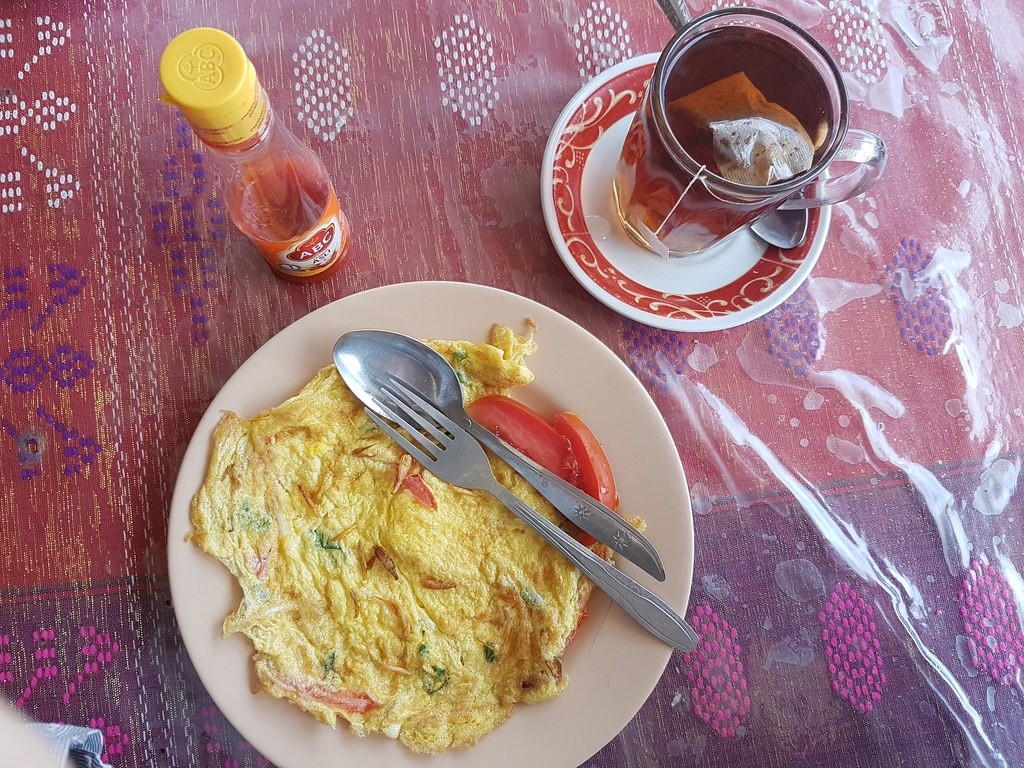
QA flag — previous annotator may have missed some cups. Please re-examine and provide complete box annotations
[611,6,889,260]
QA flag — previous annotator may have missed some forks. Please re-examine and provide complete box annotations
[364,377,701,655]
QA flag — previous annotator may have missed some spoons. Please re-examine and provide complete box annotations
[332,328,668,584]
[656,0,809,250]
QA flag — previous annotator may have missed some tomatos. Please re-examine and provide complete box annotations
[465,395,581,489]
[554,412,617,545]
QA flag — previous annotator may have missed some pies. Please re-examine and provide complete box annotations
[183,322,649,751]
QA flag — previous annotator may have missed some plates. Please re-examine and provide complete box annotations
[539,49,832,334]
[165,281,696,768]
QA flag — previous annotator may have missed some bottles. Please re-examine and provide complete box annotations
[159,26,352,286]
